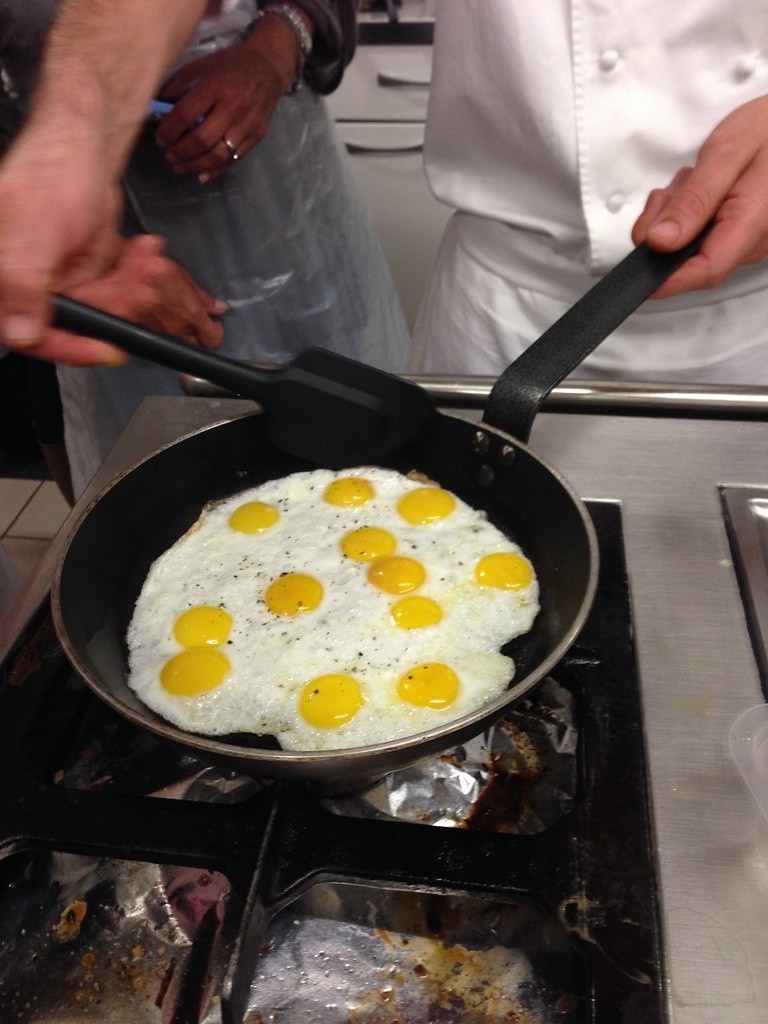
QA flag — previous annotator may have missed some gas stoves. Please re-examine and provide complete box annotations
[0,373,768,1024]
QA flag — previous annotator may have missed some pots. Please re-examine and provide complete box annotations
[53,221,713,784]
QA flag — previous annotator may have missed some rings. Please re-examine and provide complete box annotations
[221,137,236,156]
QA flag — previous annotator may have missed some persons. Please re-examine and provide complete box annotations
[0,1,412,498]
[0,0,768,386]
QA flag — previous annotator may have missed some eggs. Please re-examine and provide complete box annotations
[125,463,542,753]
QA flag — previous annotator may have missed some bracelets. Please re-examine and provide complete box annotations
[266,4,313,95]
